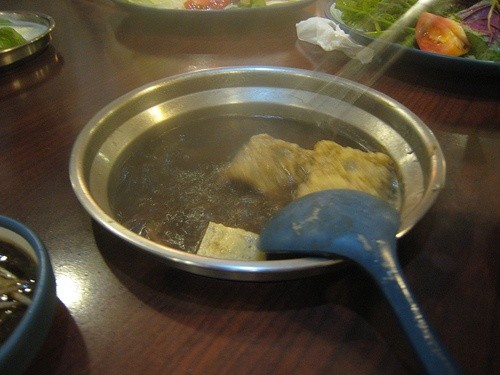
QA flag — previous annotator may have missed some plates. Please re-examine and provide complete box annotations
[0,45,65,102]
[0,10,64,66]
[116,1,315,30]
[324,0,500,81]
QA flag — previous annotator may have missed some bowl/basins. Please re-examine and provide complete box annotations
[0,215,57,375]
[69,66,445,281]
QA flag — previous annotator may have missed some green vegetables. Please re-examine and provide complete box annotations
[332,0,500,62]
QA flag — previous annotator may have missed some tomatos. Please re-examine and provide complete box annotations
[415,12,471,56]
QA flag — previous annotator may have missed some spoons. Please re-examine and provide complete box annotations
[257,188,465,375]
